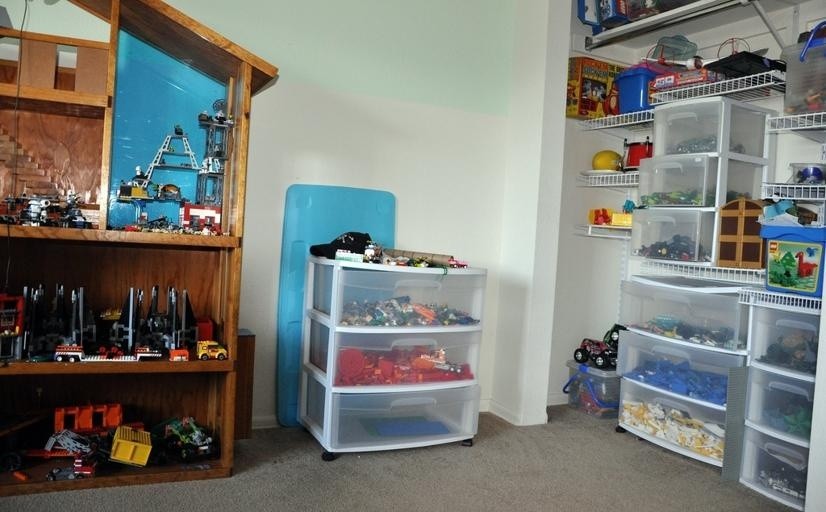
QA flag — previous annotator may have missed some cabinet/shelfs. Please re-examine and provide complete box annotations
[1,0,279,500]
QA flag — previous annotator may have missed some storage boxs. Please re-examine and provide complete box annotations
[299,257,488,459]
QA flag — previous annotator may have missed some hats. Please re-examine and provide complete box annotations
[310,232,371,259]
